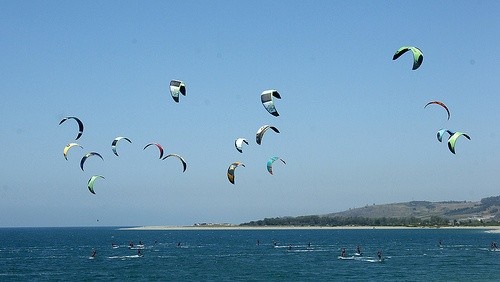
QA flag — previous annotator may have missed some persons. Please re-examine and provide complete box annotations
[377,252,382,259]
[92,248,96,256]
[290,244,291,248]
[342,249,346,257]
